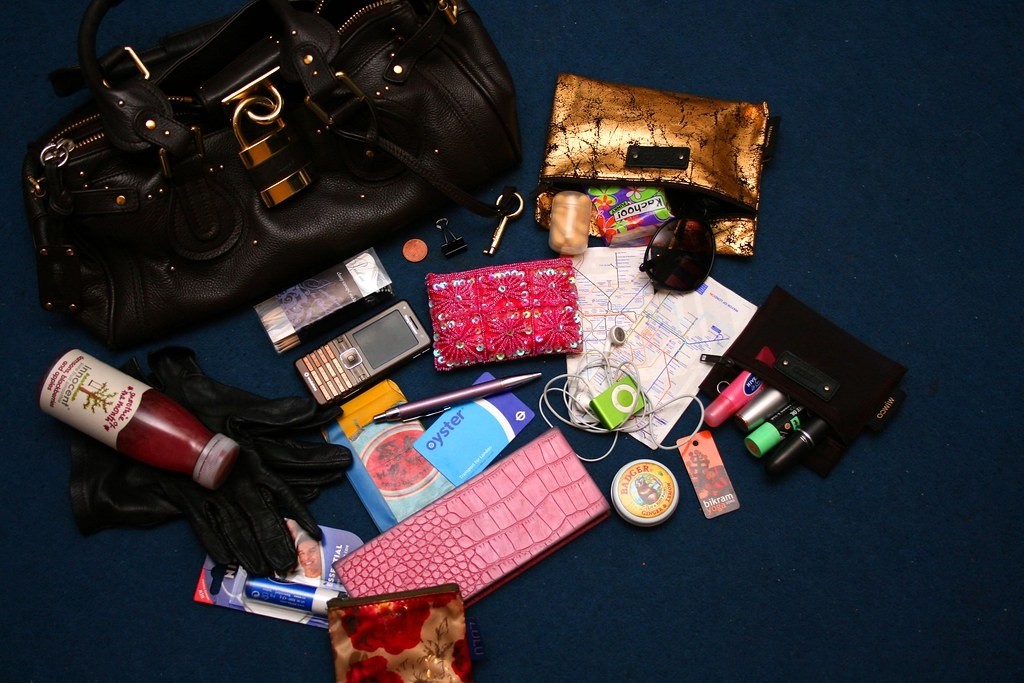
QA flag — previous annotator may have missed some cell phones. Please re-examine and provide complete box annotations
[295,300,432,408]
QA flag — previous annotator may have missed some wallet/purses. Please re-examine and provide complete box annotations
[329,583,483,683]
[424,259,582,371]
[332,427,610,611]
[535,74,780,258]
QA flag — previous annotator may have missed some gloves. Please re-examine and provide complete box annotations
[72,347,352,579]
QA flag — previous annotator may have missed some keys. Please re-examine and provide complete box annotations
[481,190,524,256]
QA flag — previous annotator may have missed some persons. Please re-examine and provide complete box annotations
[289,532,323,589]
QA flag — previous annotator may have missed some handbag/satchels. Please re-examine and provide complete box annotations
[21,0,525,353]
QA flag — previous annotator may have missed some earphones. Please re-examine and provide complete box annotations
[603,326,625,360]
[575,393,593,413]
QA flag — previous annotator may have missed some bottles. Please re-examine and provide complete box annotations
[36,347,239,493]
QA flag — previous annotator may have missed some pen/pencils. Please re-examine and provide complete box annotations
[373,371,543,426]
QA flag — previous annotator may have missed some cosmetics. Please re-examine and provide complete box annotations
[737,386,790,434]
[705,369,765,426]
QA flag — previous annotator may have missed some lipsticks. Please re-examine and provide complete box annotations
[243,574,348,619]
[768,415,831,475]
[742,400,812,458]
